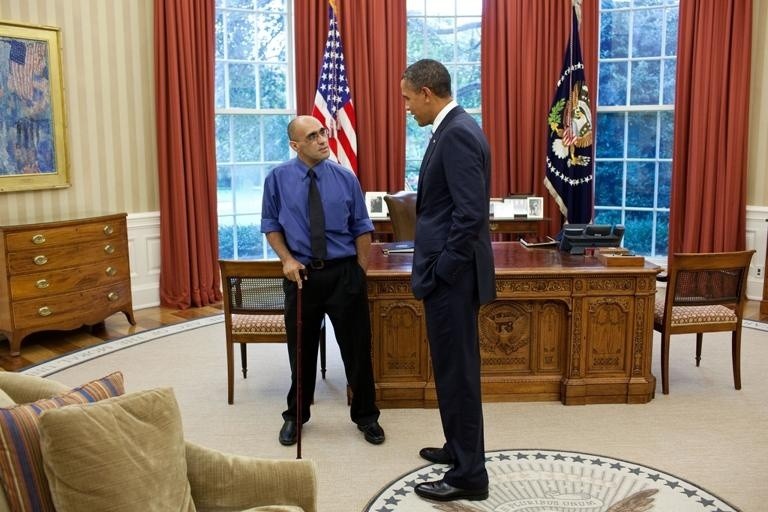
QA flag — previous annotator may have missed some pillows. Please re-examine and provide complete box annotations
[0,371,127,512]
[35,386,197,512]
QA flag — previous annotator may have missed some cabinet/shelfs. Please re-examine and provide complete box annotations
[345,241,657,405]
[0,211,137,358]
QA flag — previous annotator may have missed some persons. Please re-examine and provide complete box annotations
[397,56,497,502]
[259,114,390,448]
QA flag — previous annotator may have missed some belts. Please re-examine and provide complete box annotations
[307,260,344,270]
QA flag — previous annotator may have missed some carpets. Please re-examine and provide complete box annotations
[8,310,767,512]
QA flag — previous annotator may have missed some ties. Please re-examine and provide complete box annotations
[307,169,327,258]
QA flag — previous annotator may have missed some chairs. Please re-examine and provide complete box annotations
[0,370,320,512]
[216,257,328,406]
[383,192,417,242]
[653,247,758,396]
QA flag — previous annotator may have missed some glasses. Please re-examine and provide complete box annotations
[293,128,328,141]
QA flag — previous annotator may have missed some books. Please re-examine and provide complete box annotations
[377,239,416,255]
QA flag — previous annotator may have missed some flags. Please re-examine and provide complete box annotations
[307,0,362,185]
[543,0,598,237]
[6,39,41,99]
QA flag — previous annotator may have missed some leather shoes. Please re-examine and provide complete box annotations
[415,480,488,501]
[358,422,384,444]
[279,421,297,445]
[420,448,455,464]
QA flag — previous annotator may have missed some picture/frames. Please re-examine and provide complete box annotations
[526,196,544,219]
[365,191,388,219]
[1,20,74,194]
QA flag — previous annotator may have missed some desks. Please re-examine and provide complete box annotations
[367,216,554,242]
